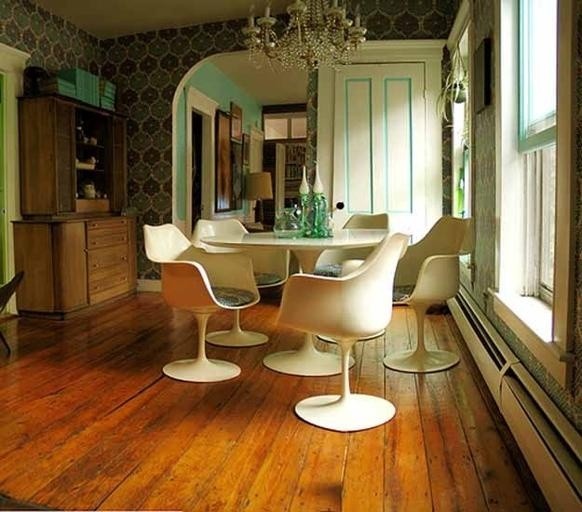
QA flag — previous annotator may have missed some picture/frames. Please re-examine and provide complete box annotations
[213,97,253,212]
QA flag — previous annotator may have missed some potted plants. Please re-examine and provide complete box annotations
[431,76,470,124]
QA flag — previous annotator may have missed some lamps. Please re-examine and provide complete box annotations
[237,0,369,75]
[242,170,275,223]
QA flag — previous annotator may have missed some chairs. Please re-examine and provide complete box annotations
[192,217,291,349]
[141,222,262,384]
[272,231,411,435]
[298,212,392,344]
[1,270,27,355]
[381,212,477,374]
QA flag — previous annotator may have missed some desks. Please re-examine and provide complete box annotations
[200,229,413,378]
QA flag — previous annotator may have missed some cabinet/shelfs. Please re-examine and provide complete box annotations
[10,216,140,323]
[13,90,129,222]
